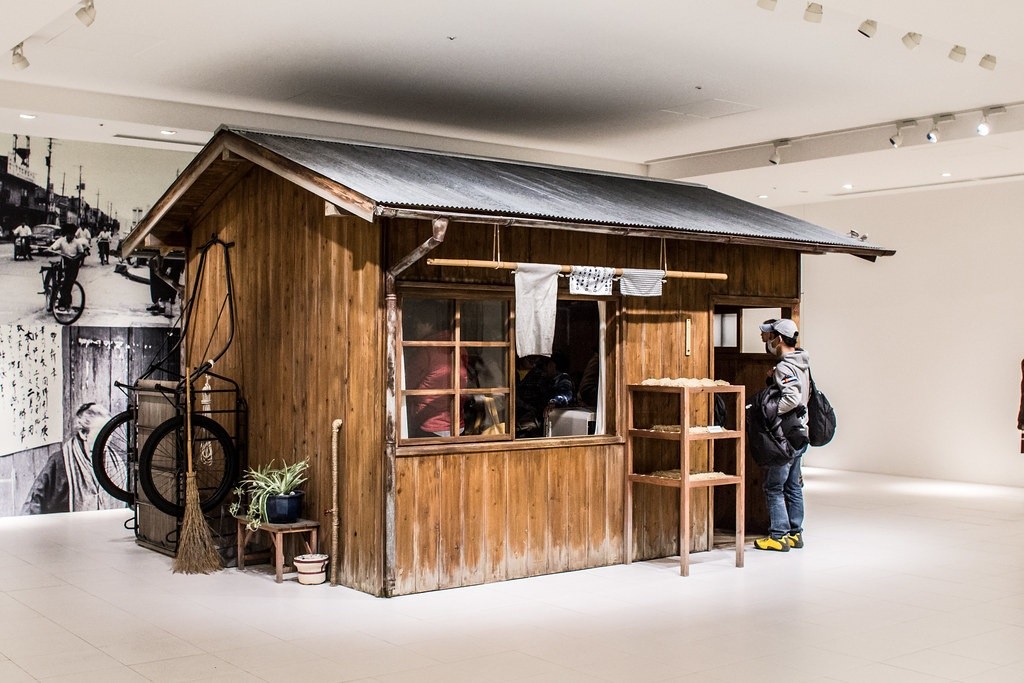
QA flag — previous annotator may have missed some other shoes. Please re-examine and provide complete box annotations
[29,257,33,260]
[87,252,90,255]
[159,306,166,313]
[107,262,109,264]
[146,304,160,310]
[98,252,100,253]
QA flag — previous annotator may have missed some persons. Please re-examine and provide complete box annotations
[574,351,599,410]
[21,402,136,512]
[11,219,185,313]
[515,351,572,438]
[404,301,469,438]
[749,318,810,551]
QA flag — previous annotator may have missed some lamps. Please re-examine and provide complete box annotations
[857,19,877,38]
[948,45,967,62]
[926,112,956,143]
[979,53,996,71]
[757,0,776,10]
[74,0,97,28]
[901,32,922,51]
[978,105,1006,136]
[803,2,823,23]
[890,120,918,150]
[768,140,790,167]
[10,43,30,70]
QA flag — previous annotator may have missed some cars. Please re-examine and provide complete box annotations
[29,224,62,256]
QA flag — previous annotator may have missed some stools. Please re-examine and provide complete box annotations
[237,517,319,583]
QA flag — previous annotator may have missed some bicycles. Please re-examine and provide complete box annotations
[37,249,86,325]
[96,240,111,265]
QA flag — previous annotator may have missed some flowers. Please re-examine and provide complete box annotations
[227,454,310,532]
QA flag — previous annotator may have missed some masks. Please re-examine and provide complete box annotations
[767,337,780,356]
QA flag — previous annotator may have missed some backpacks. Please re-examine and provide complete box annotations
[806,367,836,446]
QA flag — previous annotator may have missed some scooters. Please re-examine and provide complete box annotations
[13,236,29,261]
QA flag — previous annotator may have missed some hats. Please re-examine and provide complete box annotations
[759,319,799,339]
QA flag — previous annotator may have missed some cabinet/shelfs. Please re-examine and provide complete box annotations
[627,382,745,488]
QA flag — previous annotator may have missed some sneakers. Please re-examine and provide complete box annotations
[754,533,790,552]
[788,533,804,548]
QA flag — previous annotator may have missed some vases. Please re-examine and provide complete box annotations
[293,550,328,586]
[257,488,306,525]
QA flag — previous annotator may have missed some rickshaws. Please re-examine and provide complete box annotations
[94,238,242,560]
